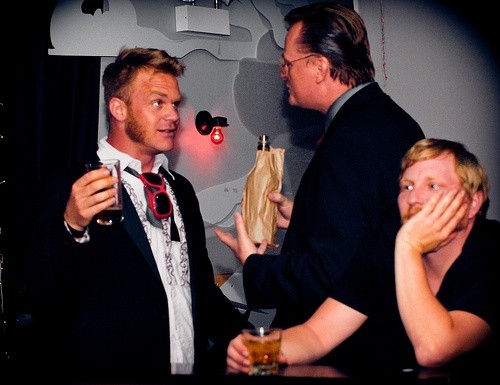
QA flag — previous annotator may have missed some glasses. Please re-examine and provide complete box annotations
[140,172,172,218]
[277,54,321,74]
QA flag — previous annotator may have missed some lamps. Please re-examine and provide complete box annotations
[195,110,230,144]
[174,0,232,39]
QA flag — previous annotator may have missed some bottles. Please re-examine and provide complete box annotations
[245,135,279,245]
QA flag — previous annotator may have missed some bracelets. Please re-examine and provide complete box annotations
[63,220,88,239]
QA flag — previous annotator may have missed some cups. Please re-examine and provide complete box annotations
[98,159,122,210]
[240,327,281,375]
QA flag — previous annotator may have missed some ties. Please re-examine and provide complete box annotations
[316,132,325,149]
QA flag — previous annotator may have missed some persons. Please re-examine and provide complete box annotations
[226,136,500,385]
[34,45,254,383]
[212,0,425,328]
